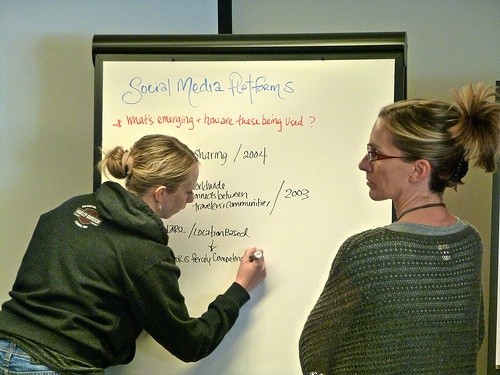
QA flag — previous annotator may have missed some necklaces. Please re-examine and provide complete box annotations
[391,201,446,221]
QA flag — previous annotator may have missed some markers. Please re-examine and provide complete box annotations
[251,250,262,261]
[86,31,407,375]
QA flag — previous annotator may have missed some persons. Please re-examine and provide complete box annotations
[296,84,500,372]
[0,134,266,374]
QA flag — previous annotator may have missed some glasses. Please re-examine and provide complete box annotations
[368,144,406,163]
